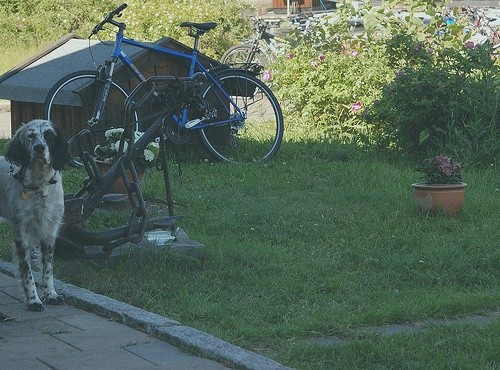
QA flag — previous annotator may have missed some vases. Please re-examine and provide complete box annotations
[91,157,147,195]
[412,181,467,218]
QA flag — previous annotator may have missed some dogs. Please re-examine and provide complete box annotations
[0,117,69,312]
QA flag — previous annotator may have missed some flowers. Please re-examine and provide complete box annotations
[415,154,463,184]
[92,126,161,168]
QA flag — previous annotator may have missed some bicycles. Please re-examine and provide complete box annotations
[41,3,284,166]
[219,12,319,95]
